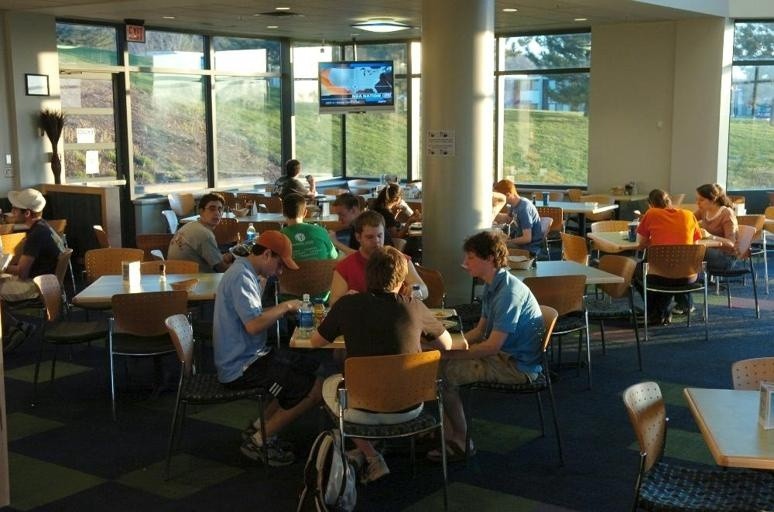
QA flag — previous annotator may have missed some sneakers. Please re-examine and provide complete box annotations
[672,306,694,314]
[346,448,363,471]
[360,456,389,484]
[4,320,36,354]
[242,420,295,467]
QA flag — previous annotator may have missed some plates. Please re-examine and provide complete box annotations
[433,313,452,318]
[440,320,457,329]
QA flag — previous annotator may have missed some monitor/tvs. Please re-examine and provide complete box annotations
[319,60,395,114]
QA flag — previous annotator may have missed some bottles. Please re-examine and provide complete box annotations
[532,195,536,205]
[299,293,314,339]
[159,263,166,276]
[314,298,325,325]
[608,190,615,205]
[246,223,256,240]
[411,285,422,300]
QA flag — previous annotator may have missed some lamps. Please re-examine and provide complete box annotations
[349,19,413,34]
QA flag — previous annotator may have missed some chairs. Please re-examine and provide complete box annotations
[492,183,774,317]
[635,244,709,340]
[0,176,447,407]
[165,314,270,478]
[464,305,562,461]
[623,382,772,512]
[521,275,592,390]
[323,351,447,511]
[732,357,772,391]
[109,290,189,421]
[579,254,642,371]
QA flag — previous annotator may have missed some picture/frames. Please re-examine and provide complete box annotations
[24,72,51,98]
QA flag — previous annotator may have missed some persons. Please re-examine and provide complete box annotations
[671,184,739,315]
[329,211,429,307]
[271,159,316,204]
[311,245,453,483]
[0,187,65,354]
[408,231,546,462]
[359,179,544,259]
[213,230,324,467]
[328,193,395,256]
[632,189,703,324]
[277,193,340,305]
[264,155,281,192]
[167,193,233,273]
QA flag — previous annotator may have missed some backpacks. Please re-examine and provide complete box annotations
[296,431,357,512]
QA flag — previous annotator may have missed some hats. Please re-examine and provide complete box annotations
[257,230,299,270]
[8,189,46,212]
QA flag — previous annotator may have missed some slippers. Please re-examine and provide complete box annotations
[428,441,474,462]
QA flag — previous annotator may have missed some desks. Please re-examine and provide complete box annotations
[684,387,773,469]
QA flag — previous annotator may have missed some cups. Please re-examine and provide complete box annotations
[628,225,637,242]
[543,193,549,205]
